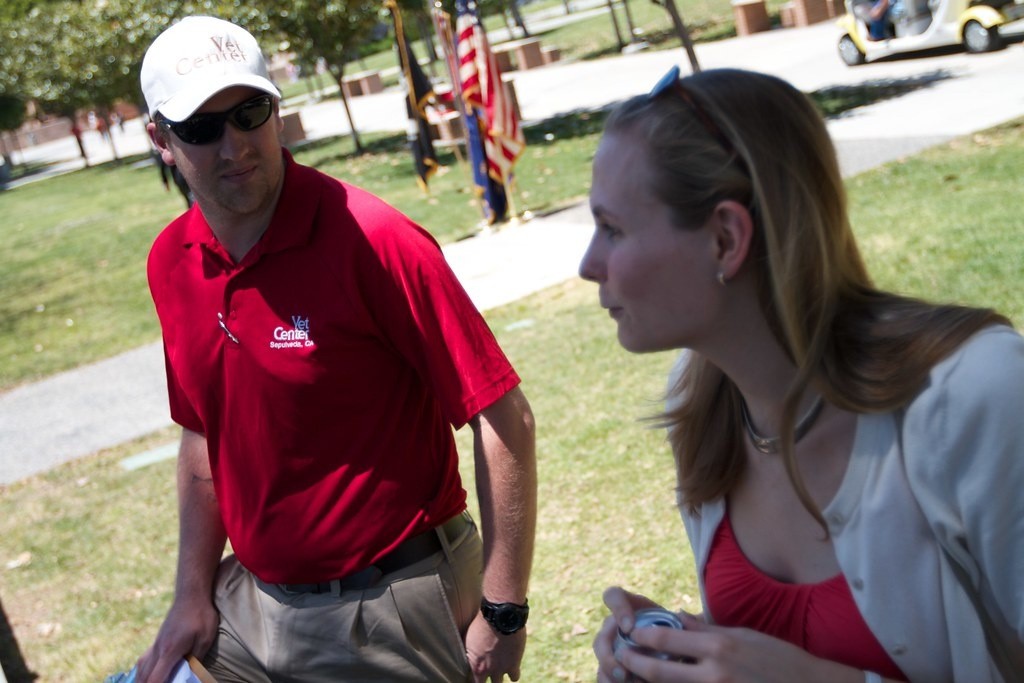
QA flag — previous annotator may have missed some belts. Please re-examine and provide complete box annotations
[276,511,476,596]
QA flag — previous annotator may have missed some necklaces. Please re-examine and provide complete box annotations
[742,393,824,453]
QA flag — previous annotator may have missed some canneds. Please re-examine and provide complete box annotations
[612,608,685,683]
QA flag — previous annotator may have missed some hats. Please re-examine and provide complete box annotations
[140,15,281,123]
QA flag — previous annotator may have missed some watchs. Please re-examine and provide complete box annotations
[481,596,529,636]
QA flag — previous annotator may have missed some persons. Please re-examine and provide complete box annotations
[581,70,1024,683]
[96,112,124,143]
[136,16,536,683]
[156,151,196,209]
[867,0,894,41]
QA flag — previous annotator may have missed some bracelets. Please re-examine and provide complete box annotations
[864,670,881,683]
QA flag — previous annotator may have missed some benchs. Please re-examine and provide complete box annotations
[489,36,543,72]
[731,0,829,37]
[280,110,305,143]
[341,72,384,96]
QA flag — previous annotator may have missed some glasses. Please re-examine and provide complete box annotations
[166,94,274,145]
[645,66,747,173]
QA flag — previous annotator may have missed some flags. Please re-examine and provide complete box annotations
[383,0,438,193]
[432,0,526,226]
